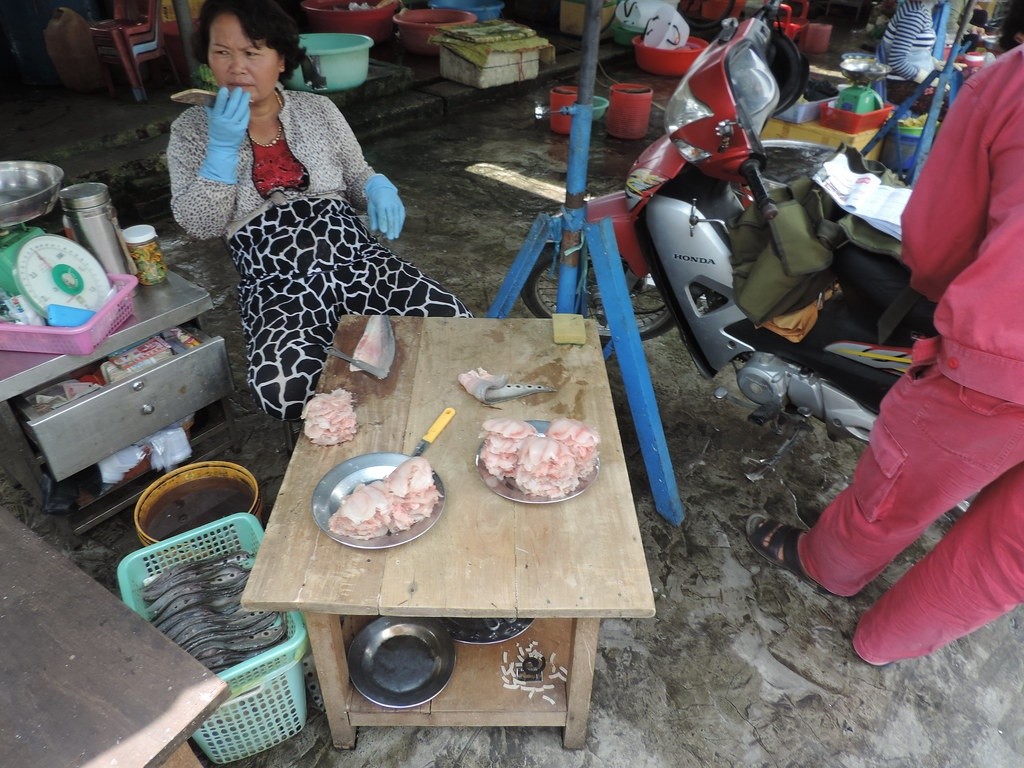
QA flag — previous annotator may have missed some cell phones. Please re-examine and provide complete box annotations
[171,89,254,108]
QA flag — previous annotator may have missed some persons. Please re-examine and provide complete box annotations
[166,1,476,421]
[743,0,1023,667]
[878,0,968,120]
[945,0,985,53]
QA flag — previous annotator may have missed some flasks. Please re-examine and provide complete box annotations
[59,185,139,299]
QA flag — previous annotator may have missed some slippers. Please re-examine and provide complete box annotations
[746,514,831,594]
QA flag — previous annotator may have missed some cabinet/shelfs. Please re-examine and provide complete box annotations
[0,269,237,536]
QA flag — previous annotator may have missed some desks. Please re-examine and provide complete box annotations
[239,317,656,750]
[0,505,232,768]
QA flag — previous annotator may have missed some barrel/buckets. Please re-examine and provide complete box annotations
[605,84,653,139]
[133,460,262,572]
[804,23,832,53]
[551,85,580,134]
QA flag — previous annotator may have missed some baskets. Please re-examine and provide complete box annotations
[114,512,305,766]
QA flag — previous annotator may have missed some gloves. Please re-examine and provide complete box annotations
[365,174,405,240]
[199,86,252,184]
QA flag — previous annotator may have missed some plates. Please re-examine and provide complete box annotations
[476,420,599,504]
[446,618,534,644]
[312,452,444,551]
[348,616,455,708]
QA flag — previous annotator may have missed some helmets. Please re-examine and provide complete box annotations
[768,25,810,119]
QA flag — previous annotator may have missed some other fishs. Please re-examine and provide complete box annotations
[141,549,288,676]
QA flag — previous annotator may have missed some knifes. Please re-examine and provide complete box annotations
[409,408,455,456]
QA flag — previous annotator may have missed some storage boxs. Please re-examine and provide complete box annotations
[560,0,617,36]
[772,94,840,124]
[759,114,883,162]
[438,44,539,88]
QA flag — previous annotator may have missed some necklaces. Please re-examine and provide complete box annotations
[249,90,283,147]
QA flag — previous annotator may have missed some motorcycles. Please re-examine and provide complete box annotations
[520,1,982,521]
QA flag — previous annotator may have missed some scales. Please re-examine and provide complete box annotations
[835,56,893,114]
[0,161,111,319]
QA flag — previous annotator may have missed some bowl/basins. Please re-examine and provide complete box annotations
[429,0,504,20]
[897,127,923,137]
[300,0,398,41]
[284,32,374,93]
[393,9,477,54]
[632,33,708,76]
[611,24,639,45]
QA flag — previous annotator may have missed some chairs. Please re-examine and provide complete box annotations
[90,0,184,105]
[744,0,809,52]
